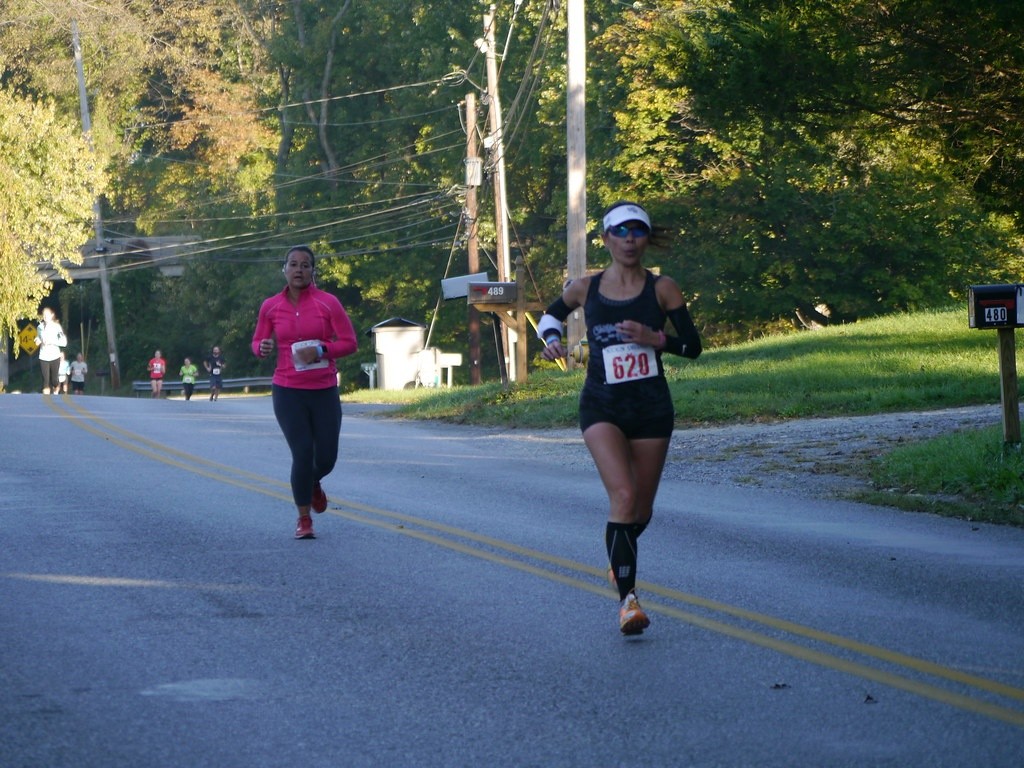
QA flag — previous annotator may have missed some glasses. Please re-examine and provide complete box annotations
[611,226,648,237]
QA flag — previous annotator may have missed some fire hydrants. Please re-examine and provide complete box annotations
[571,333,590,369]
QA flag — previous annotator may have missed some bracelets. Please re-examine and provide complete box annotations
[546,334,560,347]
[316,346,323,358]
[652,329,665,349]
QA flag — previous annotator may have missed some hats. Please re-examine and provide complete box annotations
[603,204,651,233]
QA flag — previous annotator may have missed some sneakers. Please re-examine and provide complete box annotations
[608,561,619,590]
[294,513,316,539]
[311,479,327,513]
[620,586,650,636]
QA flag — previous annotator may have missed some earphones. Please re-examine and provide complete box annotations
[282,268,285,272]
[313,271,315,275]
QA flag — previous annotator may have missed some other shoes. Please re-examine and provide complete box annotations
[209,398,213,401]
[42,388,50,394]
[53,386,59,395]
[214,399,217,401]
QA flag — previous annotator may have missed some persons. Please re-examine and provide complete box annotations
[250,245,357,540]
[34,307,88,395]
[538,200,703,636]
[178,357,199,401]
[146,349,165,399]
[203,345,226,401]
